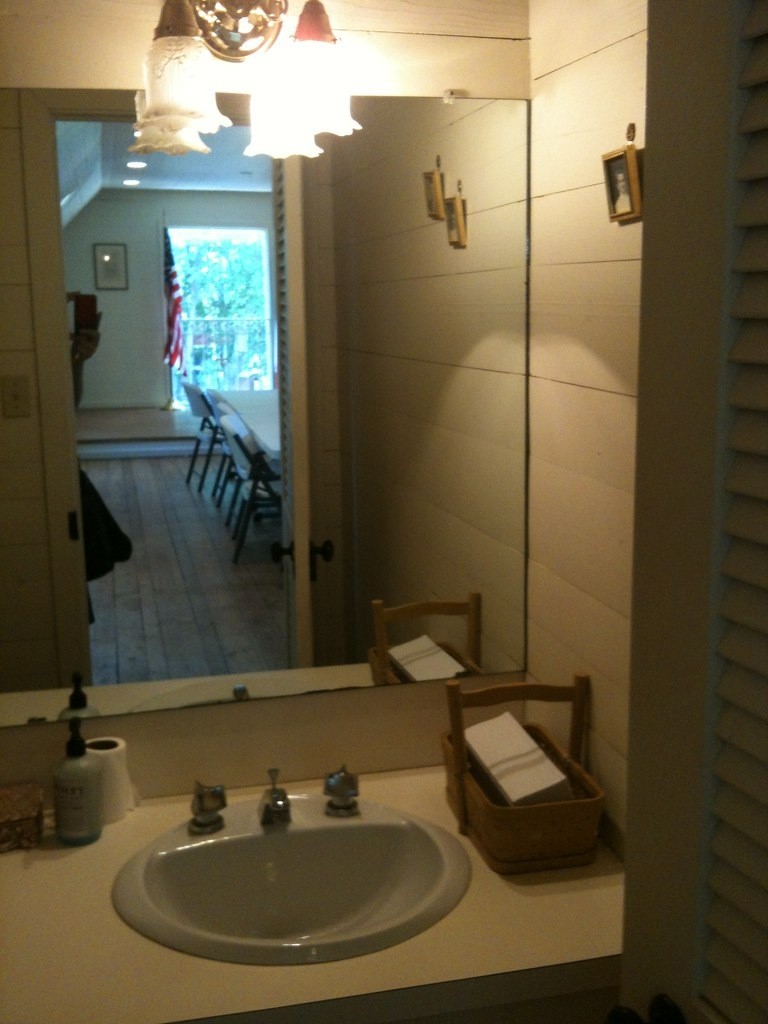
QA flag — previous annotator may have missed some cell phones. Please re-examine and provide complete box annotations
[74,294,97,334]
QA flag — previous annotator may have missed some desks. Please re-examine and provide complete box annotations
[206,388,282,461]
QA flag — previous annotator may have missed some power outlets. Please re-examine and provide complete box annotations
[0,374,31,419]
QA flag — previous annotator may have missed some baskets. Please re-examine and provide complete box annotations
[368,593,481,685]
[440,671,605,875]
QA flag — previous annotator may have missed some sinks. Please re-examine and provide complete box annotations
[113,796,472,964]
[132,678,328,710]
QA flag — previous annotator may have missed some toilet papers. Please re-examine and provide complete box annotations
[87,736,142,823]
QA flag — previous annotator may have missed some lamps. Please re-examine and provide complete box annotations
[140,0,364,137]
[126,90,325,159]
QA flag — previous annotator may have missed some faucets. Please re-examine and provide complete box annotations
[232,683,252,699]
[257,765,290,828]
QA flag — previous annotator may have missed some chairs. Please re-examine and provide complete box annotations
[205,388,266,509]
[217,413,282,565]
[182,381,228,493]
[213,401,283,528]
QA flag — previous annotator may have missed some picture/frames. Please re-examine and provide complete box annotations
[442,193,467,248]
[599,143,643,224]
[422,170,445,221]
[92,242,129,291]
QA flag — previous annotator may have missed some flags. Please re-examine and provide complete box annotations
[164,228,188,377]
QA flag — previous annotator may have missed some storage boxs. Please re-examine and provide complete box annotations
[0,782,44,850]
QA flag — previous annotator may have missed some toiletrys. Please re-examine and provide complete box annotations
[47,717,101,848]
[58,671,101,718]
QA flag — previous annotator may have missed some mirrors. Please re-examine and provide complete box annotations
[0,88,531,730]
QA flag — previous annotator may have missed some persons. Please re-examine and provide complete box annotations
[67,291,124,619]
[428,180,460,244]
[614,169,633,216]
